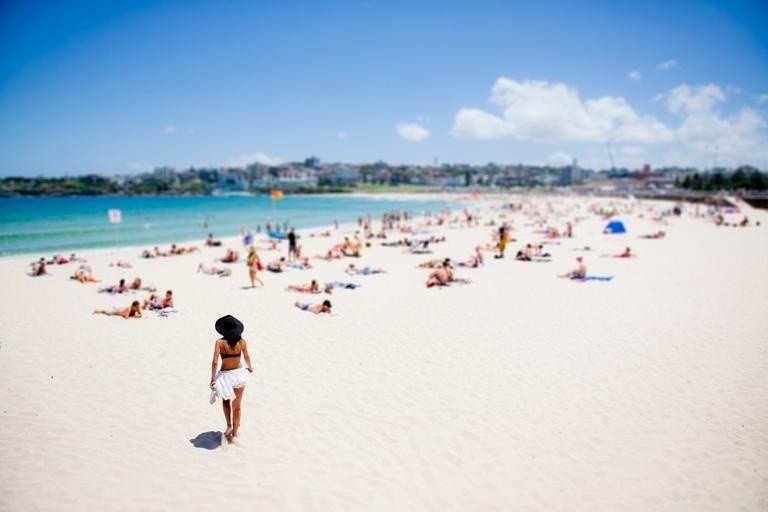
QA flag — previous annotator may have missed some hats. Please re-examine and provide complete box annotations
[215,315,244,337]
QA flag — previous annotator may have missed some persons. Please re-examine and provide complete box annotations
[313,190,636,286]
[139,220,310,288]
[26,254,175,319]
[287,281,332,313]
[638,188,748,237]
[210,315,253,443]
